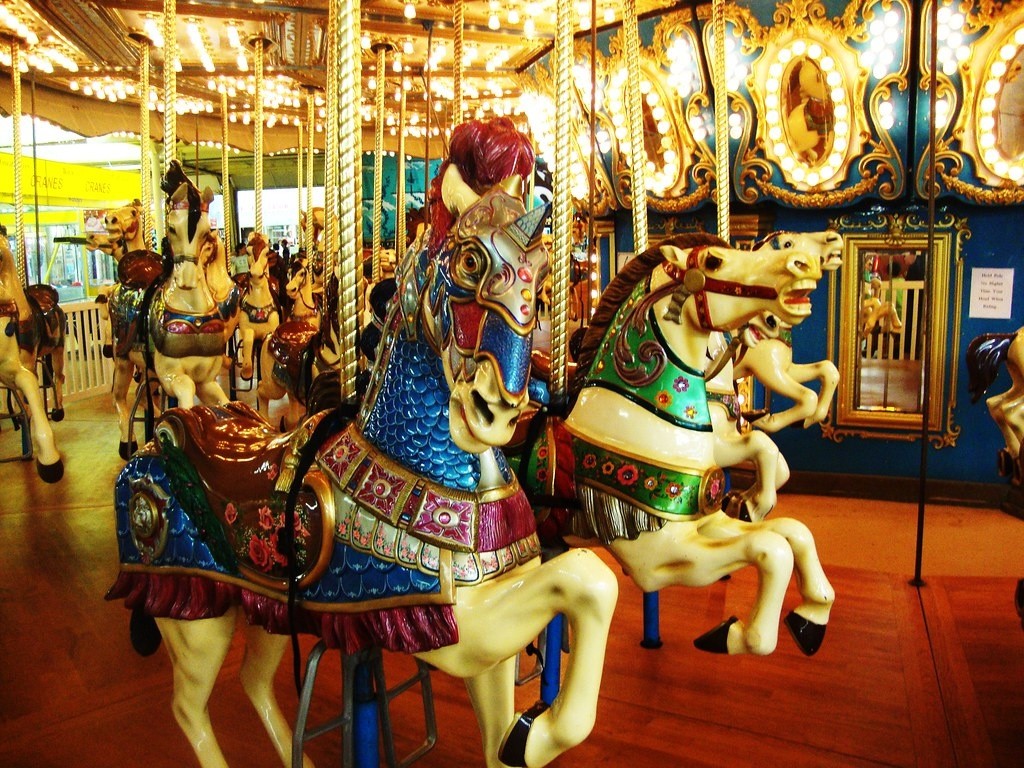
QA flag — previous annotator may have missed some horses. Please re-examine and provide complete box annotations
[0,116,1024,768]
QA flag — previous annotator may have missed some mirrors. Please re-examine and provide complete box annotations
[817,214,972,450]
